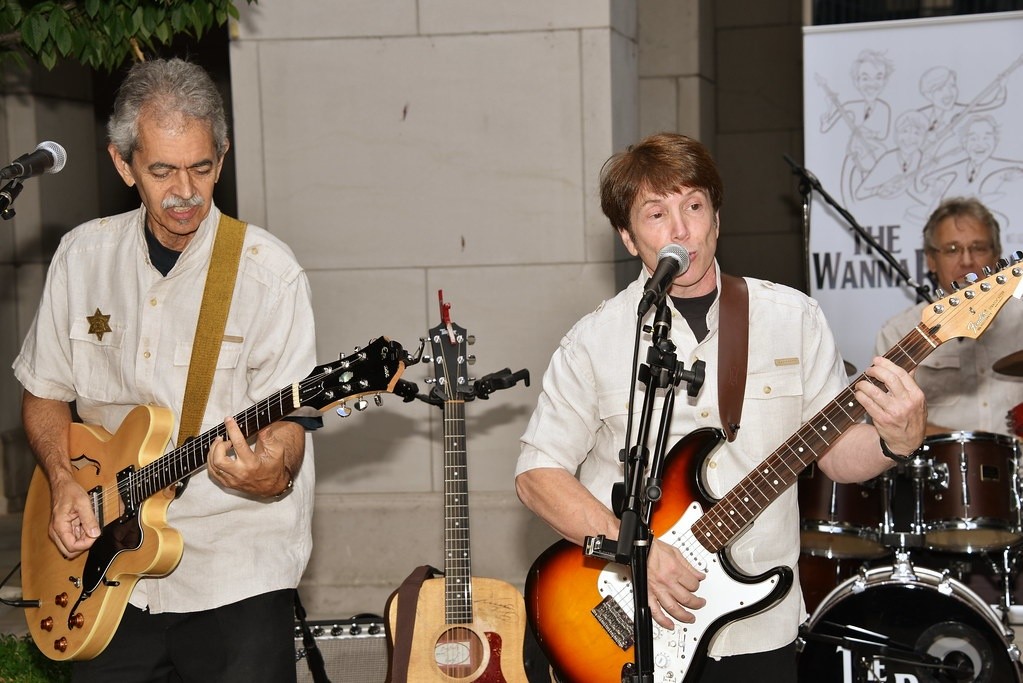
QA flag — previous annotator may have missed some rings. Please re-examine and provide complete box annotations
[218,470,223,476]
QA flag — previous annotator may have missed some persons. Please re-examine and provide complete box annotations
[875,198,1023,476]
[514,133,929,683]
[12,58,316,683]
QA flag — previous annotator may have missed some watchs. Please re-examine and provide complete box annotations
[880,438,924,463]
[275,467,293,498]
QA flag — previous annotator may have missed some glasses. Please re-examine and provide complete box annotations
[930,241,994,257]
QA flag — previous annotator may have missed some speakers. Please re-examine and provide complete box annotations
[293,617,389,683]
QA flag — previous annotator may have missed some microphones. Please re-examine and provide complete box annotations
[638,244,690,317]
[0,141,68,180]
[952,659,974,680]
